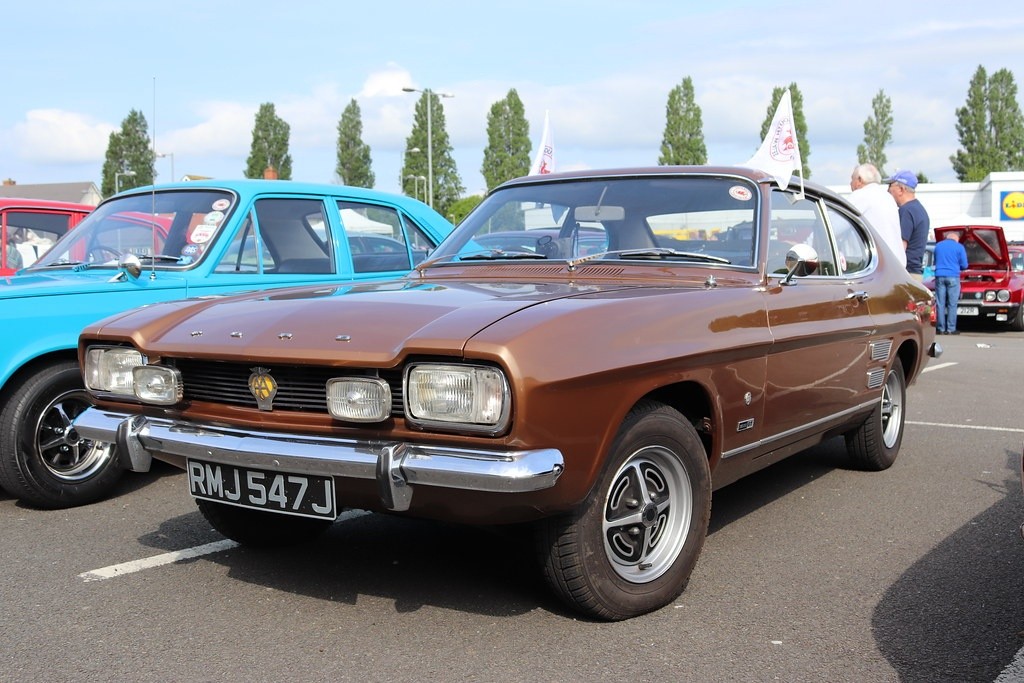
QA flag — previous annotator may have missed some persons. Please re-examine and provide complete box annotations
[882,170,929,283]
[933,232,968,335]
[846,163,907,268]
[0,227,23,270]
[13,227,51,244]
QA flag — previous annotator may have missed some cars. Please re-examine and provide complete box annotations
[0,197,188,276]
[215,228,415,272]
[471,231,608,259]
[924,224,1024,332]
[73,164,941,622]
[0,179,498,507]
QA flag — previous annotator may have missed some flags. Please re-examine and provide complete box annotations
[530,119,555,176]
[745,91,802,192]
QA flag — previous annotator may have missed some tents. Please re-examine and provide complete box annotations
[311,208,394,239]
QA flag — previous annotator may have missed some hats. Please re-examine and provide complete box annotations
[882,170,918,189]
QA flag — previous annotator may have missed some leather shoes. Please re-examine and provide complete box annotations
[936,331,945,335]
[947,330,960,335]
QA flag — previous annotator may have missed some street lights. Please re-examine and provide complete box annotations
[400,148,420,196]
[408,174,418,200]
[418,175,427,205]
[402,86,456,208]
